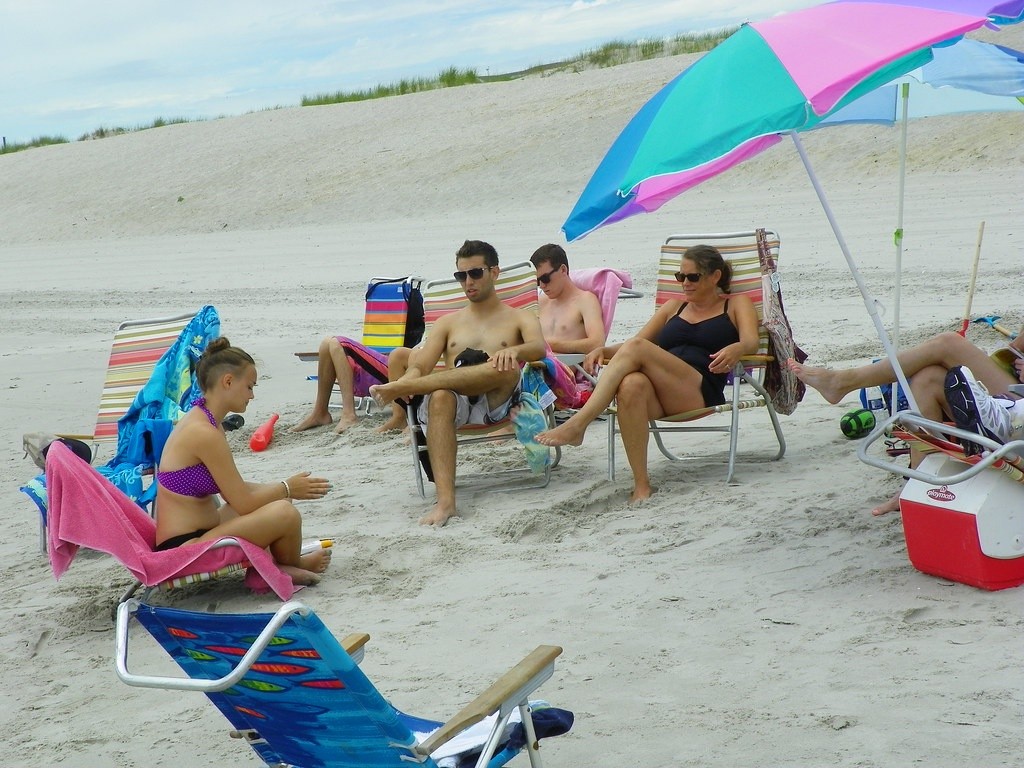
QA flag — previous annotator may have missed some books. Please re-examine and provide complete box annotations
[980,342,1024,380]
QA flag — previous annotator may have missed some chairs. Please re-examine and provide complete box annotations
[20,304,221,555]
[857,409,1024,486]
[589,227,787,486]
[294,275,426,418]
[44,440,293,620]
[115,598,575,768]
[555,266,646,447]
[406,260,562,504]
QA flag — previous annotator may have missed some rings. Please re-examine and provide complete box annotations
[1016,370,1020,374]
[727,365,731,369]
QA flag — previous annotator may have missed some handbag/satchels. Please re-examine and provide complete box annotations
[758,230,808,415]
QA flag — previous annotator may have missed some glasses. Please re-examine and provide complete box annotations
[454,266,494,282]
[674,272,705,283]
[771,272,779,292]
[537,269,555,286]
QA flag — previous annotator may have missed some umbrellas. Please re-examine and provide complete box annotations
[559,0,1024,436]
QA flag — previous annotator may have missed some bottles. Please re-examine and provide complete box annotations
[249,413,279,452]
[867,386,887,424]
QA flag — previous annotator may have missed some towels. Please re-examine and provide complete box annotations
[506,265,633,477]
[19,304,220,525]
[44,441,308,603]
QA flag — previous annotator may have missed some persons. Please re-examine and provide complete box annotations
[368,241,547,528]
[361,244,605,433]
[294,336,390,436]
[156,337,333,587]
[786,324,1024,516]
[533,246,758,505]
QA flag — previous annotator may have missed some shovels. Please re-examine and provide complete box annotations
[957,221,986,338]
[970,315,1017,340]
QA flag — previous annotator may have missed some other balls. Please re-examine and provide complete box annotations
[838,408,877,440]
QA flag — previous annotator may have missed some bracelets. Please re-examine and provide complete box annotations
[282,479,292,498]
